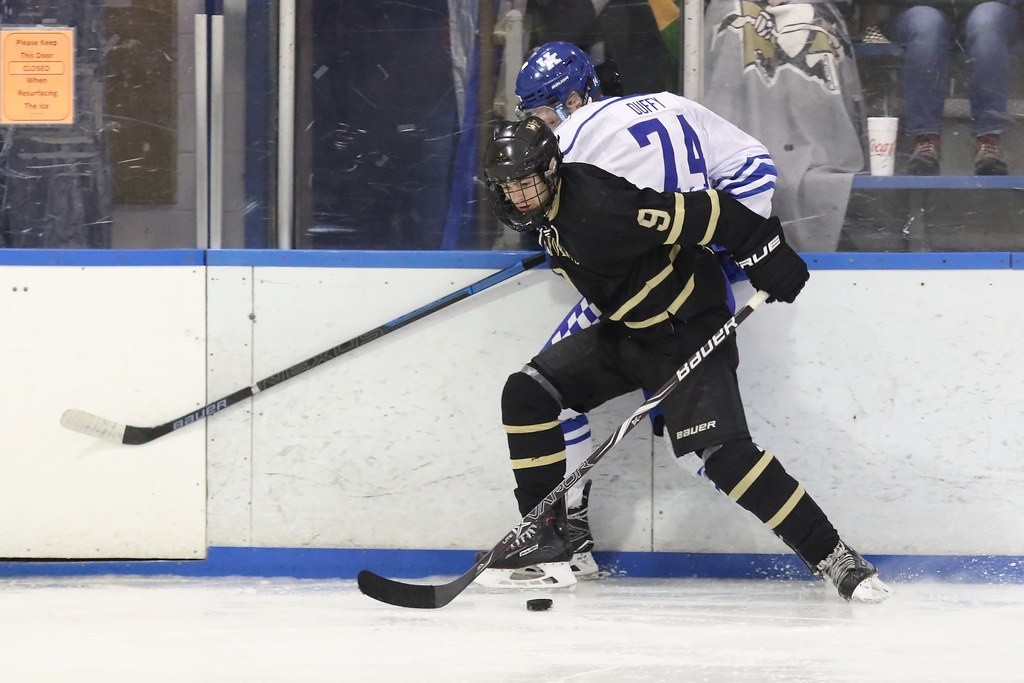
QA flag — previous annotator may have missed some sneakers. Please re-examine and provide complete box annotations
[473,500,580,590]
[906,134,941,174]
[974,134,1009,176]
[816,538,890,604]
[565,478,599,577]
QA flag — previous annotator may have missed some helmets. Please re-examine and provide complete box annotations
[514,41,603,133]
[482,115,563,234]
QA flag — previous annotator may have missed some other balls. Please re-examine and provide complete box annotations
[525,598,553,613]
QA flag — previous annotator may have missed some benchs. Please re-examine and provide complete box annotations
[849,42,1024,249]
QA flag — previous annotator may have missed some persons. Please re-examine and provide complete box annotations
[873,0,1024,177]
[482,116,894,603]
[541,0,669,96]
[514,40,777,580]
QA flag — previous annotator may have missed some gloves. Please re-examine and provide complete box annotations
[734,215,810,303]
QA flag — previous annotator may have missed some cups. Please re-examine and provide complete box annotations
[866,116,900,177]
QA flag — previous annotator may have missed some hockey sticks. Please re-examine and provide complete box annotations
[57,249,547,448]
[356,286,772,610]
